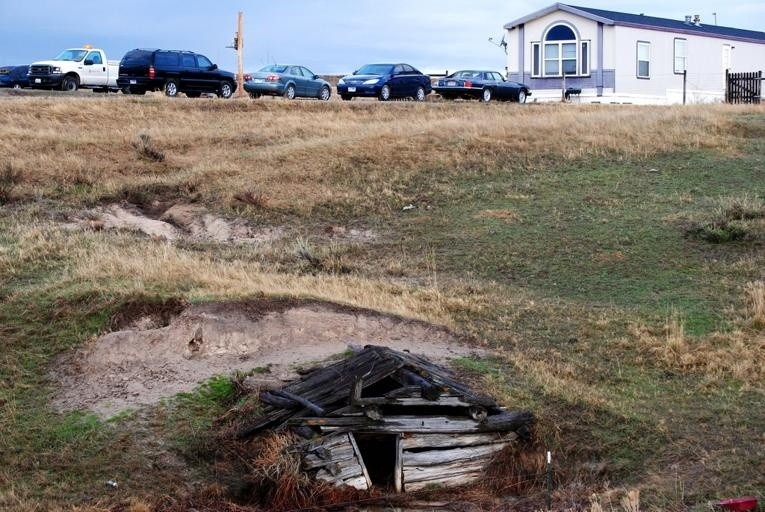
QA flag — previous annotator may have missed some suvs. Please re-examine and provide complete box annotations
[114,47,238,99]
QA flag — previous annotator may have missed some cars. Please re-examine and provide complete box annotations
[431,68,532,104]
[244,63,334,100]
[335,61,435,103]
[0,63,32,88]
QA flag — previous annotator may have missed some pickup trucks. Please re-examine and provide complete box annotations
[26,43,125,94]
